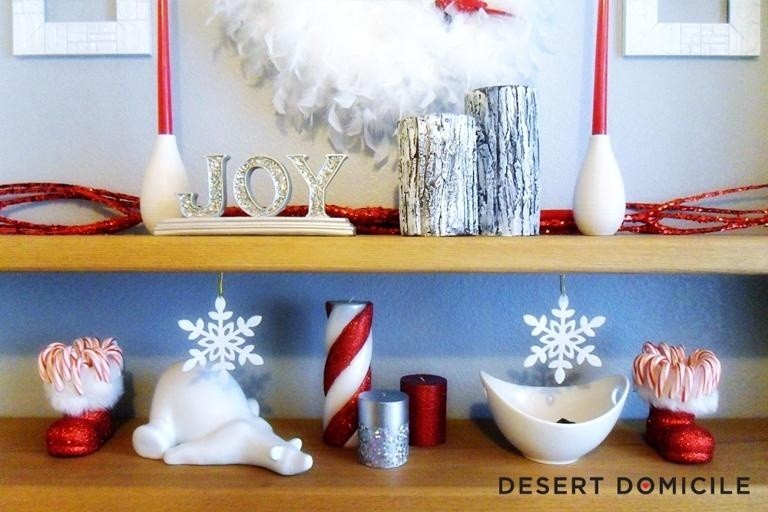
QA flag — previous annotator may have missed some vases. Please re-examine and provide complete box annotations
[570,135,627,238]
[138,129,193,237]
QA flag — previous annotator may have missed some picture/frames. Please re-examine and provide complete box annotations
[621,0,762,62]
[10,2,154,61]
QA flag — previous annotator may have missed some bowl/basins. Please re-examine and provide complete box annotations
[476,369,630,469]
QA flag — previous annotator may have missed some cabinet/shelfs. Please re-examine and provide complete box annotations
[0,220,767,510]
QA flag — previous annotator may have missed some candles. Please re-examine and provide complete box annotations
[324,288,374,453]
[400,373,450,450]
[357,388,411,468]
